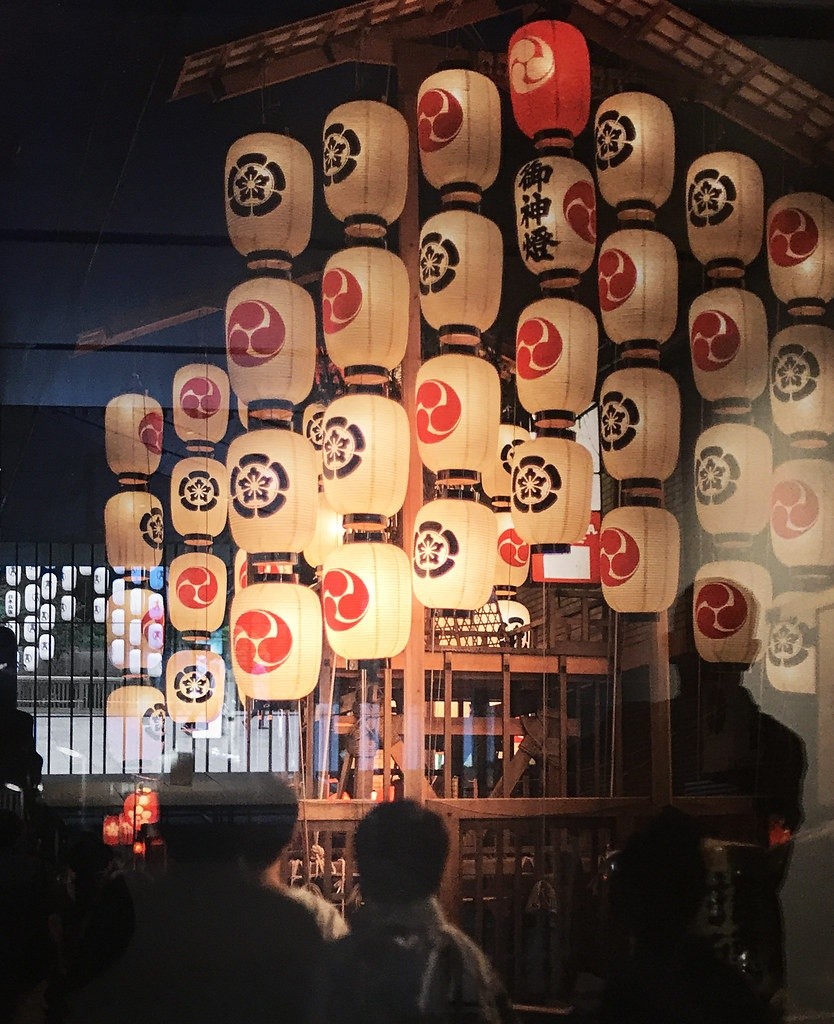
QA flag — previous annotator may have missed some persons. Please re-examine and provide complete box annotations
[1,575,809,1024]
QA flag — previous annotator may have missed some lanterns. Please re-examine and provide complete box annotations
[0,562,164,677]
[105,18,833,850]
